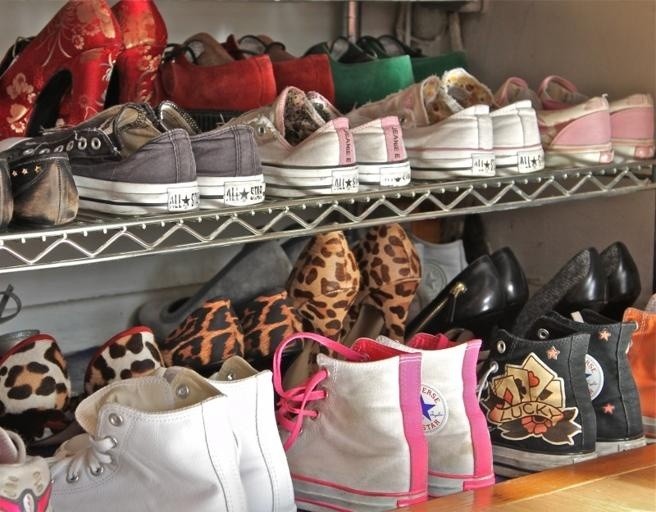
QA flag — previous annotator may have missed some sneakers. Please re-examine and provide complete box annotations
[343,75,494,179]
[406,233,468,323]
[306,90,411,187]
[535,75,655,158]
[441,67,544,167]
[216,86,359,196]
[0,102,200,216]
[150,101,266,208]
[495,77,614,163]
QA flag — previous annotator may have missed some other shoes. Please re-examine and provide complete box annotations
[0,153,80,227]
[160,33,467,132]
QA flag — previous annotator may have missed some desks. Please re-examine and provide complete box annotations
[388,450,656,512]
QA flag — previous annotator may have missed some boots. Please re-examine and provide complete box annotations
[0,286,656,512]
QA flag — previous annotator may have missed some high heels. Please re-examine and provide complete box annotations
[519,242,643,333]
[403,246,529,339]
[286,229,422,359]
[1,0,168,139]
[135,224,292,344]
